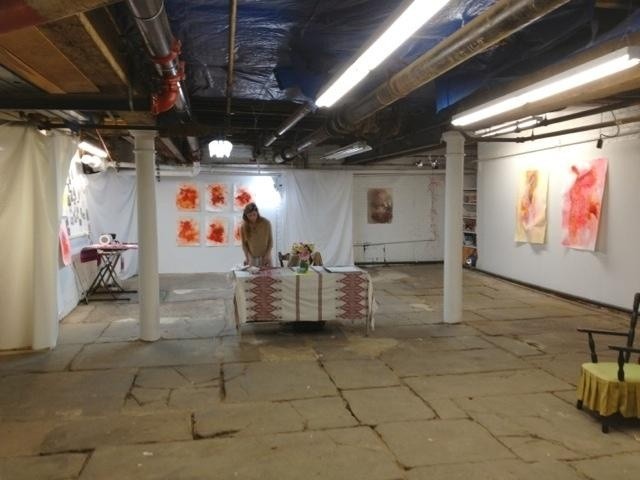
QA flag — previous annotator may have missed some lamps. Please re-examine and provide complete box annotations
[208,113,234,158]
[310,1,640,163]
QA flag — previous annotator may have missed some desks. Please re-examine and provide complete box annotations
[79,244,136,300]
[232,266,374,338]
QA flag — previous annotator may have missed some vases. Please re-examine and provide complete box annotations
[299,260,309,274]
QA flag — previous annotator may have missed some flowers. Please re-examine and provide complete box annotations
[292,241,316,263]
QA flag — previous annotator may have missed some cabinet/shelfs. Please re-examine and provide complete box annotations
[463,189,477,270]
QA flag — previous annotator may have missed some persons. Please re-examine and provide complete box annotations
[241,203,273,267]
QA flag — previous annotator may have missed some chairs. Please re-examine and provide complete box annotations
[576,292,639,435]
